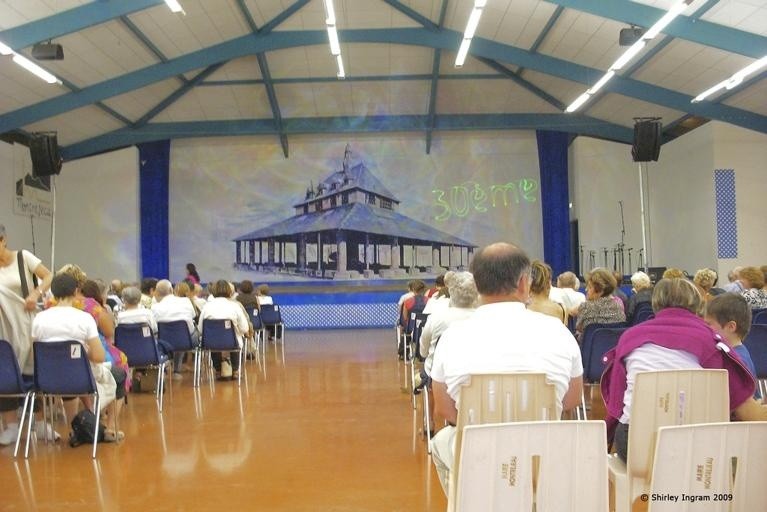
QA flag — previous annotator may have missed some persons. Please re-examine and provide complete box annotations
[0,224,62,448]
[28,263,286,446]
[395,259,766,463]
[428,238,585,501]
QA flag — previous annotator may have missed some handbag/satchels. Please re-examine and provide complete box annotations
[69,409,106,447]
[220,356,233,377]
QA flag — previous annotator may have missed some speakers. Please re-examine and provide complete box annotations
[29,130,63,177]
[631,121,662,162]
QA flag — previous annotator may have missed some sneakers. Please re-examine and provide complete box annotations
[103,430,125,442]
[34,422,61,442]
[0,423,20,446]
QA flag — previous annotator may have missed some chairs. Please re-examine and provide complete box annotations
[0,305,285,459]
[396,303,767,512]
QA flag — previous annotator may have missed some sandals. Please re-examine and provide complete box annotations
[133,376,141,391]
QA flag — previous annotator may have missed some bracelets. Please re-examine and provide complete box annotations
[36,286,45,296]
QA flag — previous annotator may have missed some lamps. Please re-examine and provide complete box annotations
[563,0,695,115]
[454,0,486,68]
[690,53,767,105]
[164,0,184,14]
[323,0,345,77]
[0,42,63,85]
